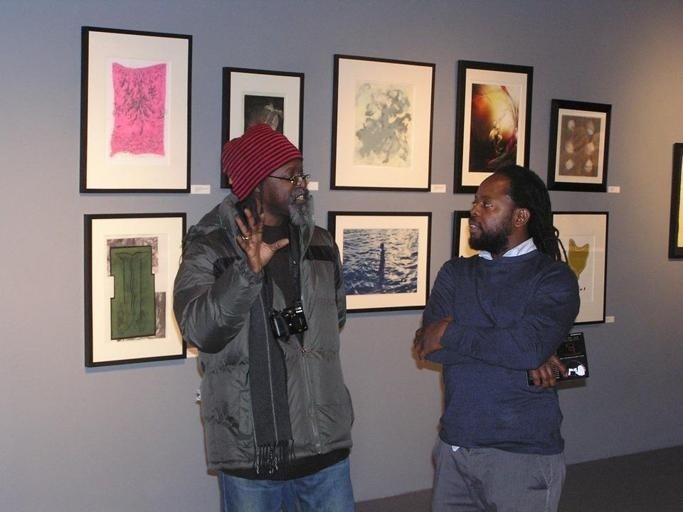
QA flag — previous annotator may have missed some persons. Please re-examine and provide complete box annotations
[171,121,355,512]
[411,161,581,512]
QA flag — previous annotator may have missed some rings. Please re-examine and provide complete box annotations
[241,235,251,240]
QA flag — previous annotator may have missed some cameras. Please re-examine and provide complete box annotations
[271,303,308,338]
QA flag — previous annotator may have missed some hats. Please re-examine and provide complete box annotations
[220,123,304,203]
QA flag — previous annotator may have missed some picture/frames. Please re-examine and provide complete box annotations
[454,60,535,193]
[668,143,683,260]
[330,54,437,192]
[327,210,433,313]
[451,208,484,257]
[546,97,612,193]
[80,25,193,192]
[551,211,609,325]
[221,66,305,188]
[84,212,190,367]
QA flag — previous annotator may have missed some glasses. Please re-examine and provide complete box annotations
[268,172,312,187]
